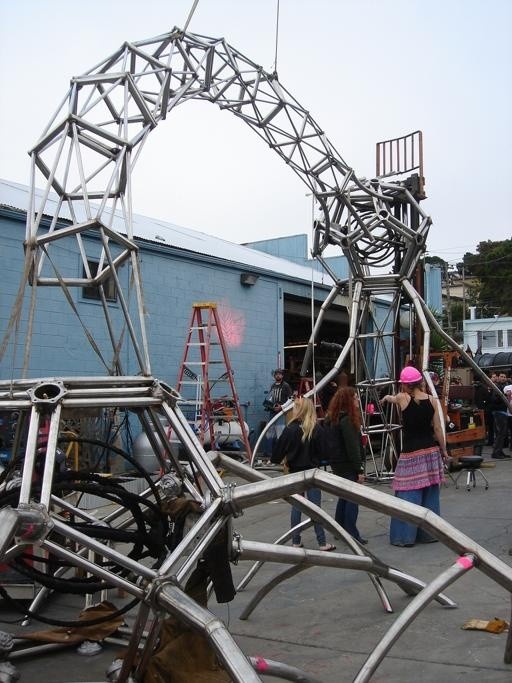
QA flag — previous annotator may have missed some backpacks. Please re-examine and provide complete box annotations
[310,412,348,461]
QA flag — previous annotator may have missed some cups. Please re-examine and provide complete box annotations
[362,433,367,446]
[468,422,477,429]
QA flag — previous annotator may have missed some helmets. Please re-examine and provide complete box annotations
[398,367,422,383]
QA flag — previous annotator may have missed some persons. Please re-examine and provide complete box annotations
[264,369,293,465]
[379,366,451,548]
[269,396,337,552]
[481,372,512,459]
[324,387,368,545]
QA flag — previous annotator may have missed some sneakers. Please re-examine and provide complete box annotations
[354,535,368,545]
[492,451,511,459]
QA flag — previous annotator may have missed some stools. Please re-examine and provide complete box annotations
[455,456,489,492]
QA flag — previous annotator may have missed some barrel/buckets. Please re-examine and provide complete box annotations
[473,352,511,368]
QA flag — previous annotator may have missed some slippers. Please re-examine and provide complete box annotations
[323,543,336,552]
